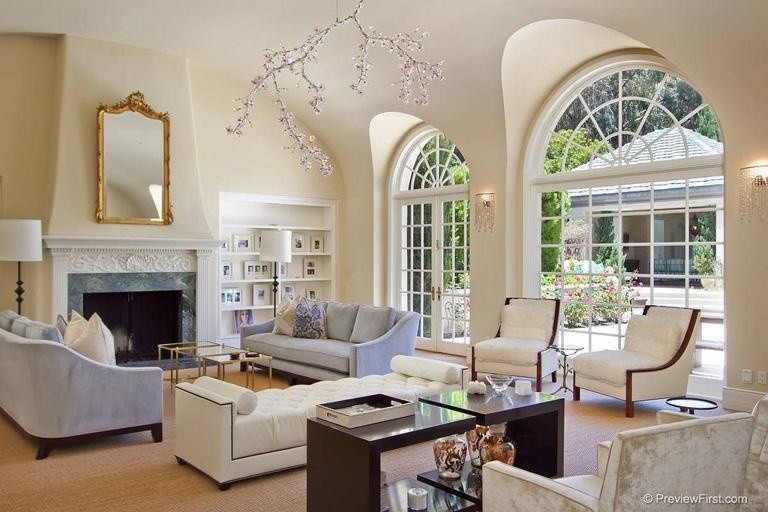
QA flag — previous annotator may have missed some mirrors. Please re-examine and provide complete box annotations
[96,89,176,227]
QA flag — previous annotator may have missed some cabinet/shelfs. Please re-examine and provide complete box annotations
[218,192,337,348]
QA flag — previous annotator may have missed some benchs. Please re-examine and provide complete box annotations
[173,352,470,493]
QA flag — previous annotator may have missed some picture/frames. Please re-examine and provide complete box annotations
[221,231,324,306]
[232,311,254,336]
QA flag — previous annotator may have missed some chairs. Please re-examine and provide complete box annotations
[481,412,755,512]
[656,394,767,512]
[570,302,701,418]
[470,296,563,394]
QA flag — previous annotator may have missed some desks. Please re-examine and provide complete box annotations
[668,396,720,418]
[304,389,569,512]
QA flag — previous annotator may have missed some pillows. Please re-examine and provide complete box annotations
[271,294,299,338]
[53,314,68,337]
[389,354,460,385]
[293,299,329,341]
[192,375,258,416]
[64,310,117,366]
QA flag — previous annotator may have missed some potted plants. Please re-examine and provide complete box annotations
[693,232,719,290]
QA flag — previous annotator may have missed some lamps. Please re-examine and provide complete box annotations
[259,228,292,319]
[732,159,768,222]
[471,192,497,234]
[0,218,46,318]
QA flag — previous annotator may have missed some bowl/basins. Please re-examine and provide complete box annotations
[486,374,514,391]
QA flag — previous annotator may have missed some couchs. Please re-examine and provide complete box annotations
[0,309,164,466]
[238,297,420,384]
